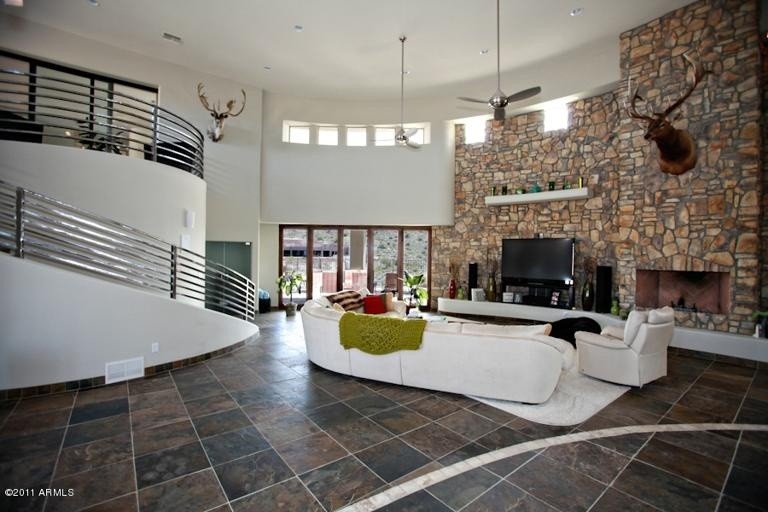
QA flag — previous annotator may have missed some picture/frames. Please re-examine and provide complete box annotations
[548,288,563,308]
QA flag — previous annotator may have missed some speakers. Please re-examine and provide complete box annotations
[595,265,612,313]
[468,261,478,301]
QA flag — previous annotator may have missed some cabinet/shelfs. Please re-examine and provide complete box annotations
[435,293,627,334]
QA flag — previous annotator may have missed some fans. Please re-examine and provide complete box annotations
[456,1,543,123]
[359,36,426,153]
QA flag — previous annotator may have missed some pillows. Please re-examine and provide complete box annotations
[646,305,675,323]
[622,308,650,347]
[325,287,394,315]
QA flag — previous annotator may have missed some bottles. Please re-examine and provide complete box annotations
[578,177,583,188]
[501,183,507,195]
[547,179,555,190]
[491,187,496,196]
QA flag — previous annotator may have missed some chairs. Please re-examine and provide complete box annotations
[571,306,677,390]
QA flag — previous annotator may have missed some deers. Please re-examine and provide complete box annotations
[624,41,706,175]
[197,82,246,143]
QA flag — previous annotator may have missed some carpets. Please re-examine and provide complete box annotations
[457,353,634,429]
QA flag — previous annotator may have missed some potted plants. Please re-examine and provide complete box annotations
[397,270,430,316]
[271,268,304,319]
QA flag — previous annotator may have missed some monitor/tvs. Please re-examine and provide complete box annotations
[501,238,575,290]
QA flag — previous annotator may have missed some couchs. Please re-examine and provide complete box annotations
[299,286,575,407]
